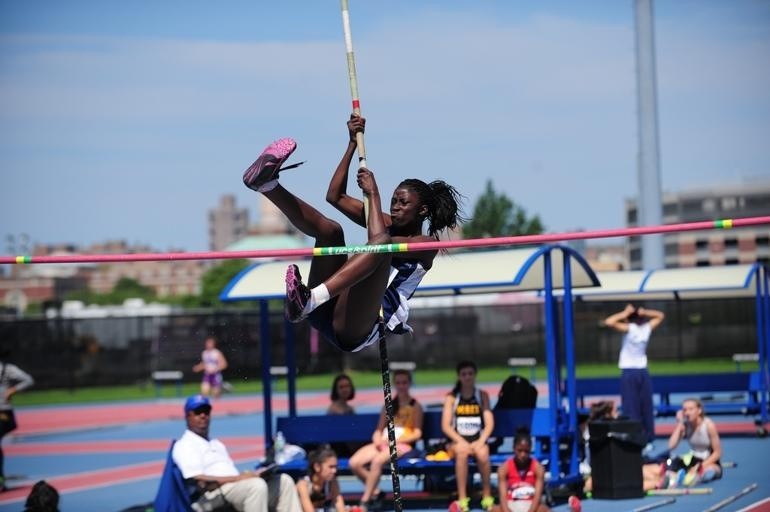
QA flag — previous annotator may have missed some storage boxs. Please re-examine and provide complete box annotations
[562,373,761,434]
[277,406,550,512]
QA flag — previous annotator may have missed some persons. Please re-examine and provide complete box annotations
[327,373,356,416]
[296,442,348,512]
[578,400,705,495]
[242,112,472,354]
[666,397,723,482]
[440,360,495,512]
[348,369,424,512]
[0,361,36,494]
[602,301,666,457]
[150,393,303,512]
[487,424,583,512]
[25,480,63,510]
[192,334,227,402]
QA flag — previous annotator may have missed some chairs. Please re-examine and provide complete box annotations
[656,463,701,489]
[569,496,581,512]
[361,490,386,509]
[285,264,313,324]
[448,497,471,512]
[481,496,495,510]
[242,138,297,192]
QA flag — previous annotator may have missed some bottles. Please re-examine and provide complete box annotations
[185,394,212,413]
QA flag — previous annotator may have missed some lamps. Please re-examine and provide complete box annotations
[173,439,210,511]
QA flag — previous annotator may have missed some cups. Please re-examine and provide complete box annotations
[194,408,210,415]
